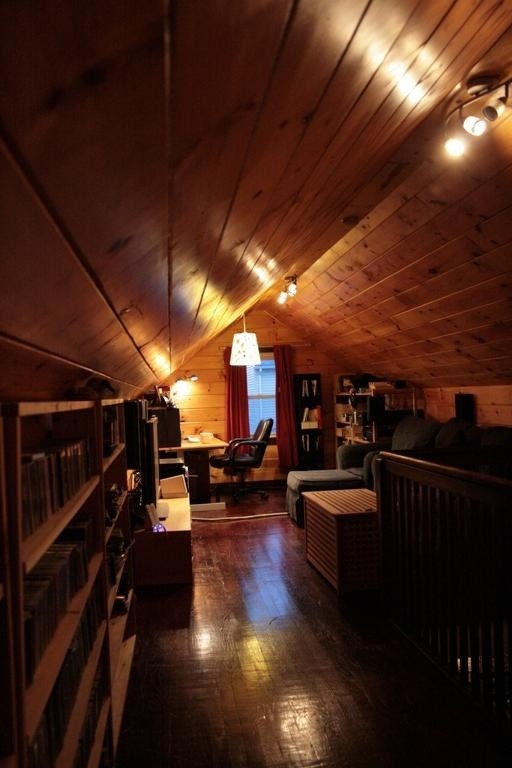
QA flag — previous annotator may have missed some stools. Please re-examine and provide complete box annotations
[287,468,361,526]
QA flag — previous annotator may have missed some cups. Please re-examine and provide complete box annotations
[199,432,213,443]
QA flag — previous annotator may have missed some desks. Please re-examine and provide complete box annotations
[136,488,193,586]
[160,428,230,505]
[300,488,378,596]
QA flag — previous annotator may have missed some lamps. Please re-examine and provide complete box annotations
[227,316,264,365]
[441,74,512,164]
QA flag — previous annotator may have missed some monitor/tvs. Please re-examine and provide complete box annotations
[145,415,168,520]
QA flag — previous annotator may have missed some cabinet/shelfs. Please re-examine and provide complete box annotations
[334,388,416,451]
[293,373,325,470]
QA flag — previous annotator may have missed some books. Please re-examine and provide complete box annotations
[301,378,321,452]
[18,435,90,542]
[101,406,120,458]
[334,380,397,447]
[21,517,90,690]
[25,575,112,768]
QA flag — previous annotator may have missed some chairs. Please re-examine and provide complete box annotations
[337,411,439,489]
[432,417,511,476]
[211,417,273,504]
